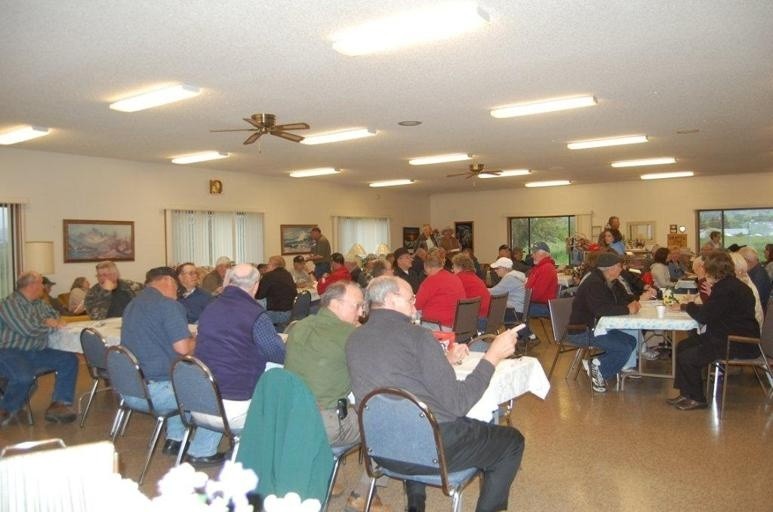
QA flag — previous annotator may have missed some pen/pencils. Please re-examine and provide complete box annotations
[512,323,526,332]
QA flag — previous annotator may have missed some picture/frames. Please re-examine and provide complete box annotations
[401,225,419,249]
[454,221,474,257]
[61,217,135,262]
[278,222,319,256]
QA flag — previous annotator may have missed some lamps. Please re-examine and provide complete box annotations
[170,148,229,165]
[25,239,55,290]
[489,97,694,192]
[286,128,472,188]
[109,86,198,116]
[476,169,532,179]
[0,119,48,147]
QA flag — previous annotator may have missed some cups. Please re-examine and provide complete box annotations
[656,305,665,319]
[565,267,577,275]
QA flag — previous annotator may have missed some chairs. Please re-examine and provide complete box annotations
[167,353,245,471]
[417,262,573,361]
[709,290,772,419]
[462,333,524,473]
[356,384,485,511]
[542,291,609,389]
[292,290,312,323]
[242,369,365,511]
[104,344,180,488]
[0,292,129,435]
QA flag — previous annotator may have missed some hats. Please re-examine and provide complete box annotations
[490,257,513,269]
[597,253,623,267]
[42,276,56,285]
[529,241,549,252]
[294,255,306,263]
[513,247,525,253]
[146,267,188,296]
[394,247,409,258]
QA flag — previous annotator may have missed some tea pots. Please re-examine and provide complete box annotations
[636,239,646,249]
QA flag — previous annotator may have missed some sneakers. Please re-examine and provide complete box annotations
[620,370,641,379]
[640,350,660,361]
[344,491,392,512]
[524,336,541,350]
[582,359,607,392]
[332,483,346,496]
[44,402,77,424]
[0,407,27,425]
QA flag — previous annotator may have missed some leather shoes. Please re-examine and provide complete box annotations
[666,395,687,406]
[163,439,189,455]
[188,453,225,467]
[676,399,708,410]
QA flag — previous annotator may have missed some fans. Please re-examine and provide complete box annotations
[208,112,309,147]
[445,156,504,181]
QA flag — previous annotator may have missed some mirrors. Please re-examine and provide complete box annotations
[624,219,658,246]
